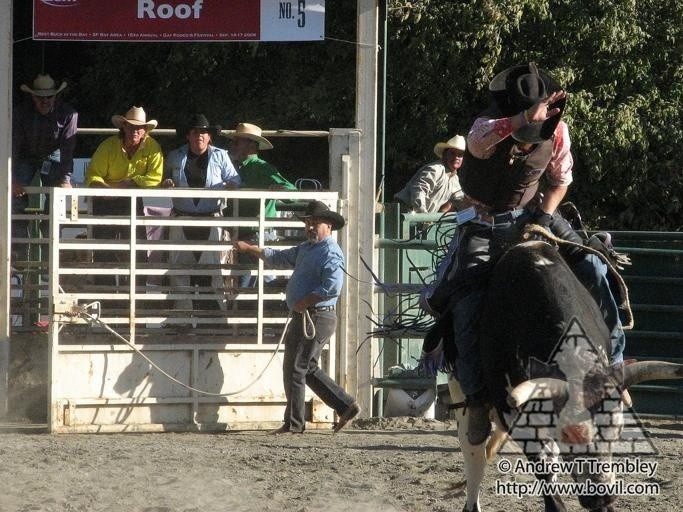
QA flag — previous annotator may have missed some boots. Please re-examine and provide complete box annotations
[610,351,634,410]
[455,355,493,447]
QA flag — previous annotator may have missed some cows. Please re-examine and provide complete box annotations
[447,218,683,511]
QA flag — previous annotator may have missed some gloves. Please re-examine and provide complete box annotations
[522,211,560,250]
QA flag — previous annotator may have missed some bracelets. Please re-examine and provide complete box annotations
[519,110,530,126]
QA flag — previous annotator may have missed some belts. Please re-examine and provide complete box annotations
[291,304,335,316]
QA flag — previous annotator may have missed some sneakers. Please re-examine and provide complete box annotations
[333,400,363,433]
[268,423,305,436]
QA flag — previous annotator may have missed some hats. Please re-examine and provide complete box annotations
[175,113,222,140]
[295,200,346,232]
[19,73,68,97]
[432,133,468,159]
[110,106,159,132]
[485,62,567,144]
[220,122,275,151]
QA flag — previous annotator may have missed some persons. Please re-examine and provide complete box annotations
[231,200,361,435]
[217,122,303,288]
[72,234,96,286]
[380,134,486,237]
[84,105,165,329]
[12,75,78,282]
[452,64,632,445]
[160,113,243,326]
